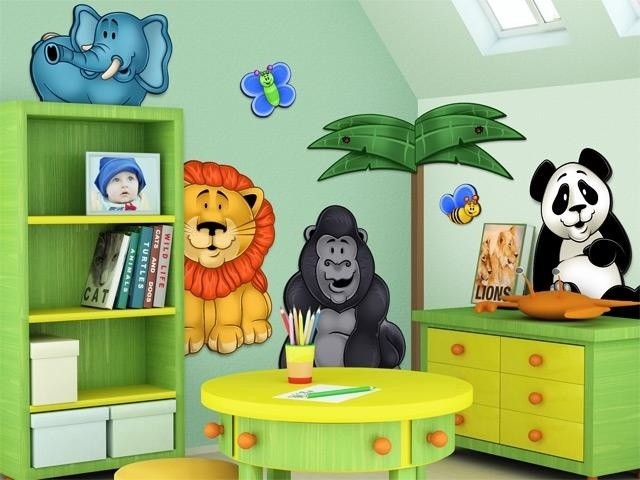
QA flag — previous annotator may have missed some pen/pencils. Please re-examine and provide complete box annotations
[305,385,378,399]
[278,303,322,346]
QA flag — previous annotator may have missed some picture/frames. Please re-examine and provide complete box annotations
[85,150,162,216]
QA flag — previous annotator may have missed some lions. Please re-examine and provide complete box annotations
[474,237,493,296]
[490,225,518,291]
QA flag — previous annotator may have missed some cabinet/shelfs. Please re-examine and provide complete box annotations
[0,102,186,480]
[410,310,640,480]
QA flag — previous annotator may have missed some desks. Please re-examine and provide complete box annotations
[199,367,474,480]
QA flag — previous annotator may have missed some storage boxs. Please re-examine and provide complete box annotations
[29,332,177,470]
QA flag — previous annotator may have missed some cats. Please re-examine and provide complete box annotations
[89,233,123,288]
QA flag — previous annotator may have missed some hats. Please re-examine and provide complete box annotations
[94,156,146,197]
